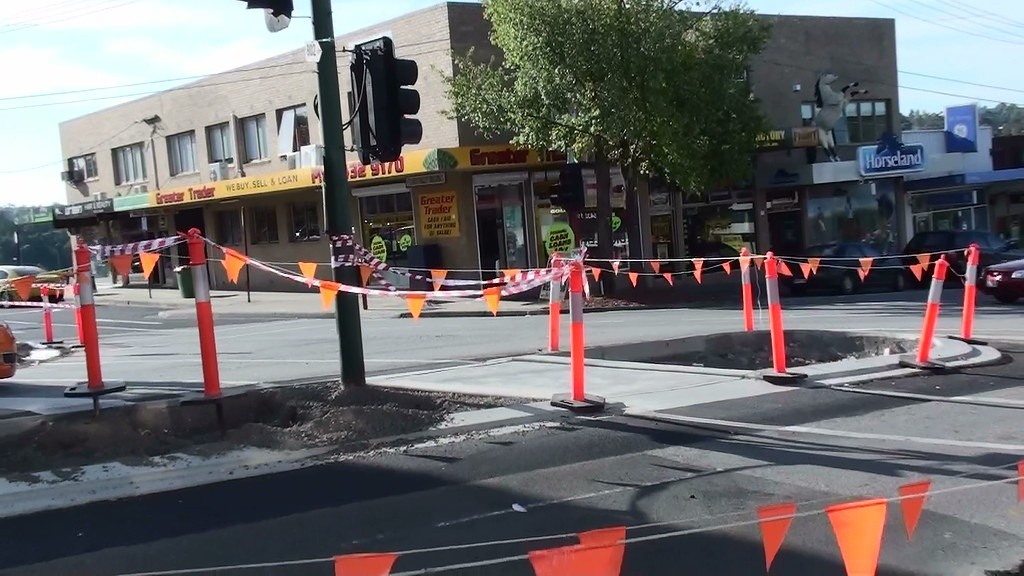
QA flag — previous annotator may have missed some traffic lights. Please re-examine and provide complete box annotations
[549,167,586,211]
[360,37,423,164]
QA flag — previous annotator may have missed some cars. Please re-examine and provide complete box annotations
[681,240,749,271]
[0,265,66,304]
[779,243,906,295]
[978,256,1024,304]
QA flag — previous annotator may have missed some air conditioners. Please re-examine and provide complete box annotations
[209,163,229,182]
[133,184,147,194]
[286,143,323,171]
[92,191,108,202]
[61,170,84,184]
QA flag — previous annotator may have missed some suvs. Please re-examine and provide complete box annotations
[901,230,1024,288]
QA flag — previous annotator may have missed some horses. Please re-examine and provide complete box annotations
[803,71,868,165]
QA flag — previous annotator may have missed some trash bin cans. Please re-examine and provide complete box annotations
[173,265,195,298]
